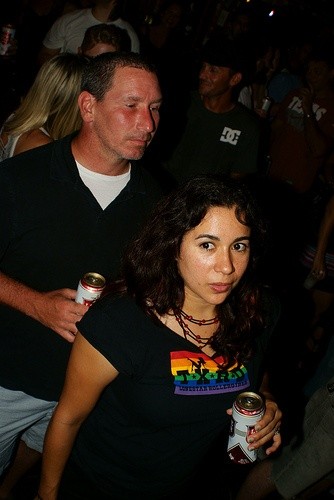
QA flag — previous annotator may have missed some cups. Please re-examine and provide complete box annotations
[304,269,321,289]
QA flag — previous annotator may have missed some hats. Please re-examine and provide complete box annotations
[197,40,234,67]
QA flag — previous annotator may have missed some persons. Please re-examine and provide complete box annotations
[236,376,334,500]
[0,51,162,499]
[1,0,279,185]
[35,176,283,500]
[241,0,334,369]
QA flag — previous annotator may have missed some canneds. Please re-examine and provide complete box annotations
[258,96,272,111]
[227,392,265,464]
[75,272,106,308]
[0,24,16,55]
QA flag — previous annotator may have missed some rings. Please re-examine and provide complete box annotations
[274,428,279,434]
[319,269,323,273]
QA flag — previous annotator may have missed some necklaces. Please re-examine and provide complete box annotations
[173,308,220,349]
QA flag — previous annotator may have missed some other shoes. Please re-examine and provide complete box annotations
[307,320,323,353]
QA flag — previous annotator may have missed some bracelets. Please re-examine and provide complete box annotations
[303,112,313,119]
